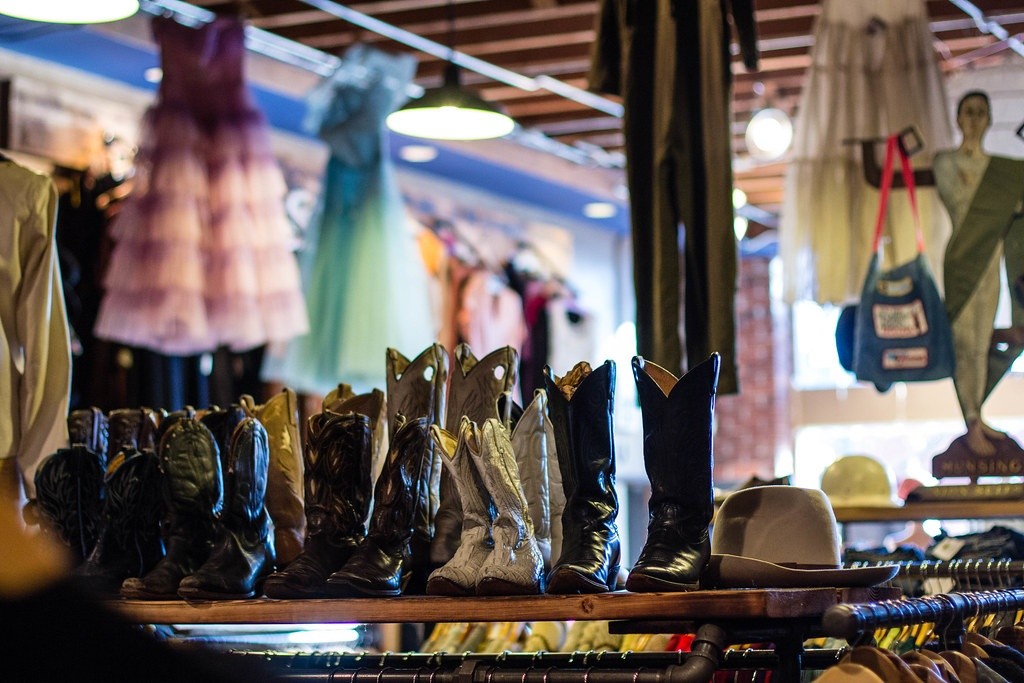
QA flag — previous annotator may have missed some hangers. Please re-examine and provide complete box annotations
[220,517,1024,683]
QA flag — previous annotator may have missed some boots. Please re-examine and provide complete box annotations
[492,388,551,586]
[529,389,566,570]
[542,359,621,595]
[429,421,494,597]
[465,418,545,597]
[33,342,450,595]
[624,351,721,594]
[429,342,518,577]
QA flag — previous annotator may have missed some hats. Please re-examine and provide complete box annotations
[711,485,899,586]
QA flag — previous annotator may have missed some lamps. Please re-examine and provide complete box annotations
[385,0,514,141]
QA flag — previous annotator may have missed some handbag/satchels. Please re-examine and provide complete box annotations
[850,135,955,379]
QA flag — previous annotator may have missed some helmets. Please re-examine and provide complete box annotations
[823,456,906,508]
